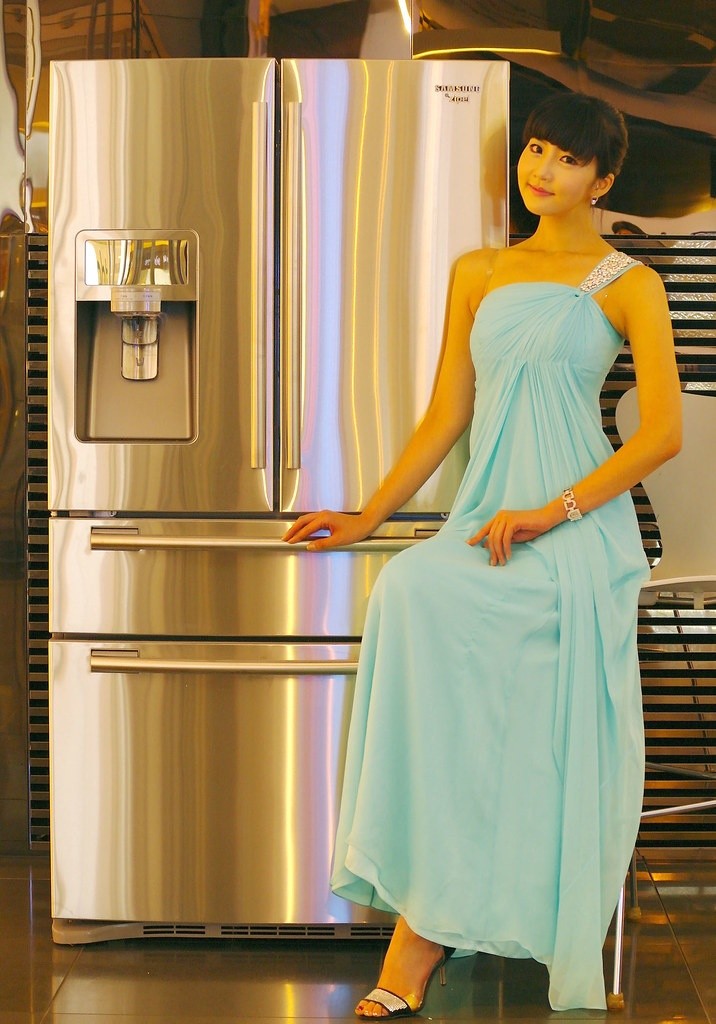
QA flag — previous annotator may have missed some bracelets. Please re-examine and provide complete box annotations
[561,485,583,522]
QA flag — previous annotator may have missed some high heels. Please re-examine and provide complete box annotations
[359,945,456,1022]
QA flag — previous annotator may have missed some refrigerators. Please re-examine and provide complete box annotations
[48,57,510,946]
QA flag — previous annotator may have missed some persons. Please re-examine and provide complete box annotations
[280,90,686,1020]
